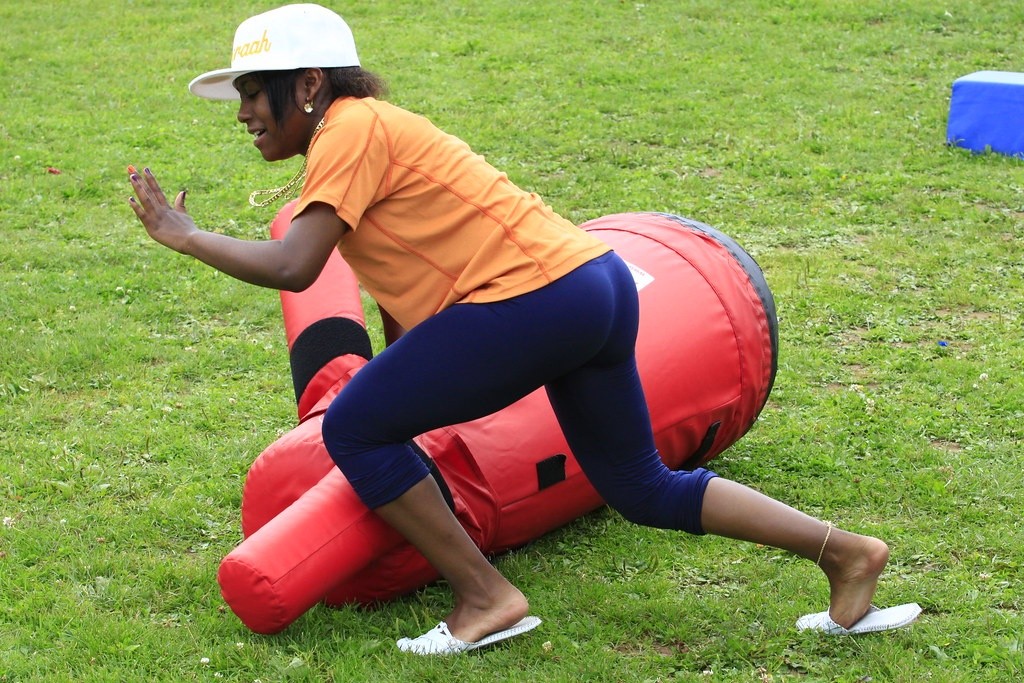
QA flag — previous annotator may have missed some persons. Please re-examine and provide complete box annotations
[127,3,921,658]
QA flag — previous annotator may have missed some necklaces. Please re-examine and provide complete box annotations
[249,115,326,208]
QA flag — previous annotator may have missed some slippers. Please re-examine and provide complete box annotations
[796,599,923,634]
[396,616,546,655]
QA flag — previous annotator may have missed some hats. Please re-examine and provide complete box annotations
[186,3,361,101]
